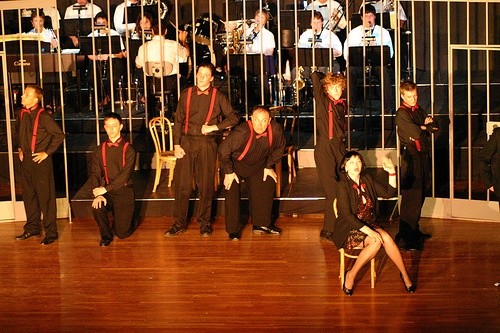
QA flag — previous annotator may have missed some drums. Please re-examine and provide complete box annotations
[189,15,219,46]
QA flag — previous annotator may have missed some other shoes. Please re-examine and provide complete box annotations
[46,104,53,115]
[40,235,58,245]
[100,233,113,246]
[200,221,212,237]
[165,224,187,236]
[406,241,424,251]
[422,233,431,239]
[228,231,240,241]
[252,223,281,234]
[16,230,40,240]
[97,94,110,110]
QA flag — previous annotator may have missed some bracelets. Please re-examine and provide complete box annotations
[389,173,396,176]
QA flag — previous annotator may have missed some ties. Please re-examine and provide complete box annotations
[319,4,327,8]
[377,0,384,2]
[365,28,374,33]
[314,34,320,39]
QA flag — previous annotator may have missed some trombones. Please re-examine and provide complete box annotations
[309,0,354,47]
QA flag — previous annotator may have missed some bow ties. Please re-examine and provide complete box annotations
[107,142,119,147]
[197,89,209,95]
[255,132,267,139]
[22,108,31,113]
[334,97,347,106]
[411,103,419,112]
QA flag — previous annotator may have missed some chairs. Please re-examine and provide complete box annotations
[147,116,177,193]
[270,106,299,184]
[146,61,178,122]
[359,149,401,220]
[332,198,377,290]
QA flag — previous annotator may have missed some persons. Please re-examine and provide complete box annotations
[133,20,191,116]
[113,0,169,55]
[219,106,288,241]
[164,60,243,239]
[305,0,348,35]
[340,4,395,63]
[86,12,125,112]
[91,111,137,247]
[237,8,275,56]
[311,66,349,241]
[27,12,61,111]
[295,11,342,60]
[358,0,408,29]
[396,80,440,252]
[130,13,164,108]
[331,151,415,296]
[15,85,66,246]
[64,0,104,46]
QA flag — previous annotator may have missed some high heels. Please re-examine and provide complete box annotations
[343,270,353,296]
[399,271,415,292]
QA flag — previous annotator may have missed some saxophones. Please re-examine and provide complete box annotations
[228,19,259,54]
[362,20,375,44]
[292,27,322,90]
